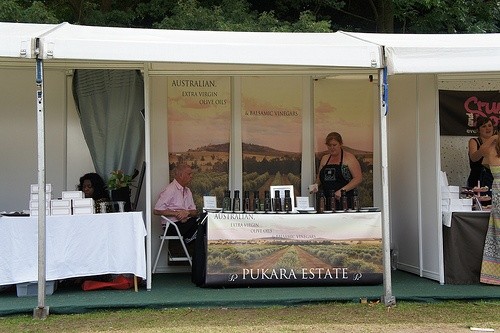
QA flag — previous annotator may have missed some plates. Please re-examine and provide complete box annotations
[203,207,379,213]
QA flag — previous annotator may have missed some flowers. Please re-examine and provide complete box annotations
[103,167,131,189]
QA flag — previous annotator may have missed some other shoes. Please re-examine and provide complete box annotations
[192,277,206,289]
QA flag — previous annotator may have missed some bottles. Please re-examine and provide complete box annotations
[340,190,348,210]
[223,190,231,211]
[274,190,282,211]
[329,189,337,211]
[265,191,271,211]
[253,191,261,211]
[243,191,250,211]
[284,190,292,210]
[233,190,240,211]
[352,189,361,212]
[319,190,325,213]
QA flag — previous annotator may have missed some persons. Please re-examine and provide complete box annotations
[470,133,500,285]
[311,132,362,210]
[153,164,206,288]
[80,173,109,200]
[466,116,493,211]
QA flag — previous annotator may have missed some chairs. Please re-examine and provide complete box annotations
[152,214,192,273]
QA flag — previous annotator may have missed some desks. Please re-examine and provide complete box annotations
[192,212,384,288]
[0,211,146,293]
[442,210,491,284]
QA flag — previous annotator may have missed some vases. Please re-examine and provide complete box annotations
[111,186,132,211]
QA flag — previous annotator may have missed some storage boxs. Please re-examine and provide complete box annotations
[16,279,58,297]
[29,184,96,217]
